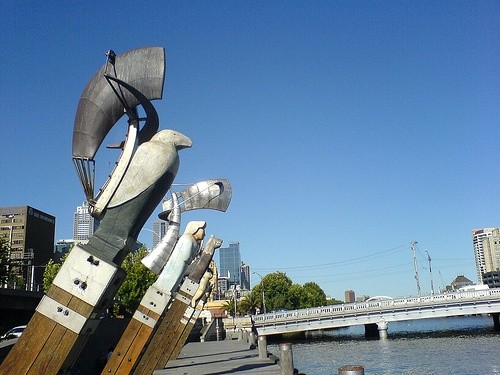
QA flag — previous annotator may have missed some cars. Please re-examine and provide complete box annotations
[267,308,289,313]
[366,296,393,304]
[3,326,27,339]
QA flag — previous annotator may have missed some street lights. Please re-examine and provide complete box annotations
[252,271,267,314]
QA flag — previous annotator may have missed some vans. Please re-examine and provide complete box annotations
[446,284,490,300]
